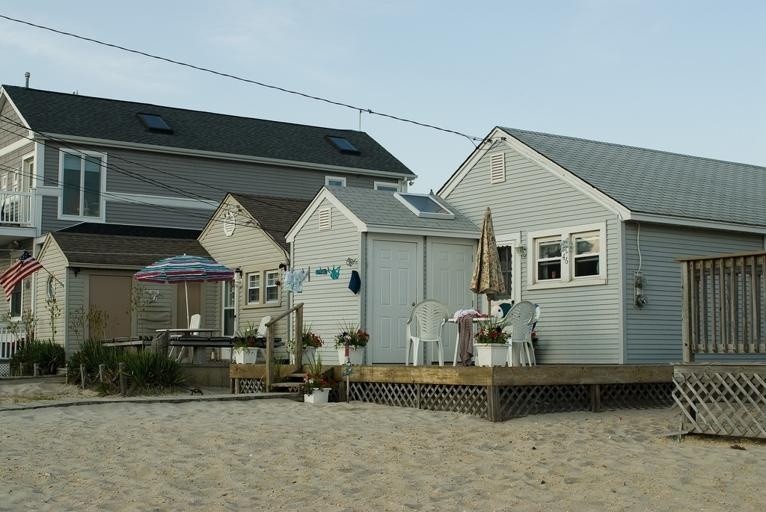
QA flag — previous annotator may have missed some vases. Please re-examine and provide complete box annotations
[290,347,317,365]
[336,346,366,367]
[303,387,332,404]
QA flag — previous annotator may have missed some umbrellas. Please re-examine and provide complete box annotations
[133,252,235,328]
[469,206,505,316]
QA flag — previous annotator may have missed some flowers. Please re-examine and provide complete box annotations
[333,316,370,349]
[298,350,334,396]
[285,319,325,352]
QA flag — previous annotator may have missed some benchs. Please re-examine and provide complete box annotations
[99,335,153,354]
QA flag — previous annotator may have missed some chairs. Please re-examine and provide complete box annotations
[404,299,538,368]
[154,313,286,364]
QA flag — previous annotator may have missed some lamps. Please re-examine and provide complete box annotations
[515,241,527,257]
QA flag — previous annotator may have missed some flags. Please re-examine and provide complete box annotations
[0,247,44,302]
